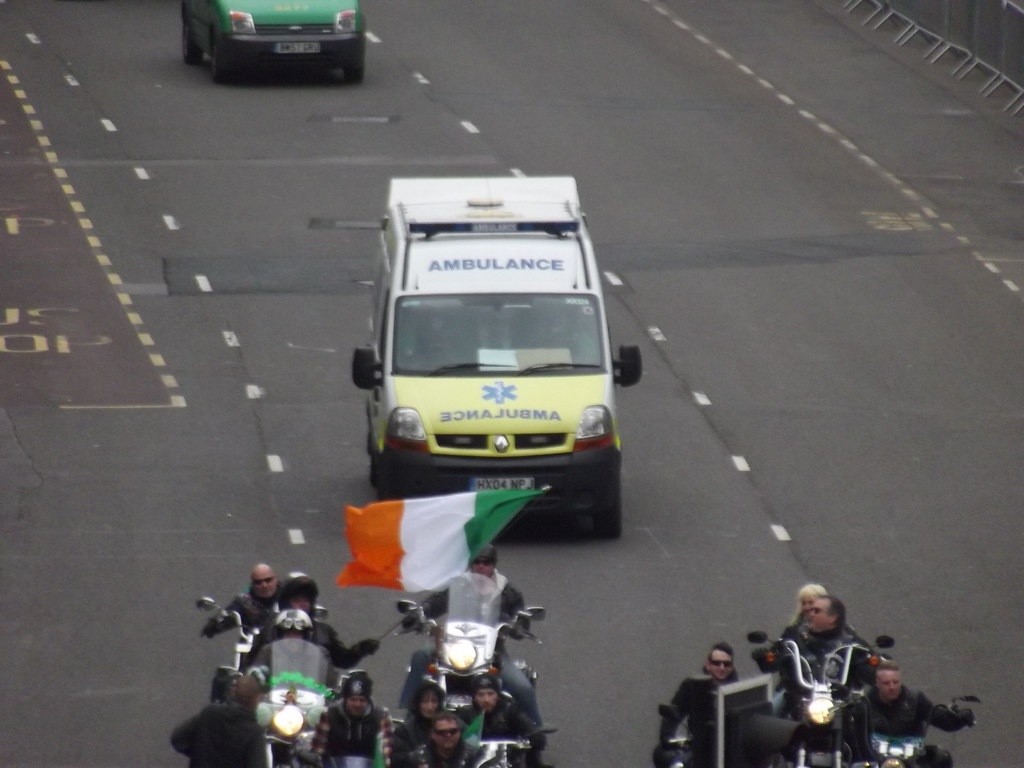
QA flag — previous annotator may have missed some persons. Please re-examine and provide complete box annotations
[659,583,975,768]
[170,542,548,768]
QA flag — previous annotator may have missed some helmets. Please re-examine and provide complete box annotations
[271,608,313,641]
[276,571,319,616]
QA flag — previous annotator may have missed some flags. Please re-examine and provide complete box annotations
[336,490,542,592]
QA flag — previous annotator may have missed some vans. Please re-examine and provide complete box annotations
[181,0,367,87]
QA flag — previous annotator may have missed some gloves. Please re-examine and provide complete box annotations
[359,639,380,655]
[402,614,417,628]
[958,708,975,727]
[751,647,768,661]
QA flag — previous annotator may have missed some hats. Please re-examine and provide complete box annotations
[471,674,499,699]
[478,544,498,567]
[343,673,373,696]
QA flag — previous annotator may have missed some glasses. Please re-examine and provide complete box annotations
[708,657,733,667]
[432,728,459,736]
[251,577,276,584]
[474,559,493,566]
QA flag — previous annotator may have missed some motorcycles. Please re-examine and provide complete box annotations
[655,630,982,768]
[194,595,391,768]
[396,571,558,768]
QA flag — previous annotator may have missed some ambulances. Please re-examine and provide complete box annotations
[350,176,643,541]
